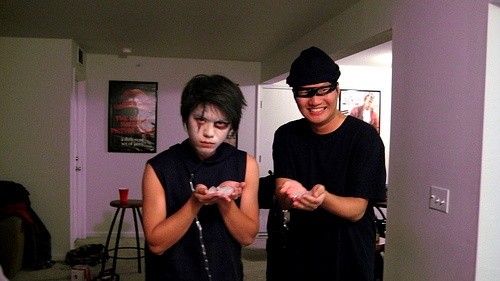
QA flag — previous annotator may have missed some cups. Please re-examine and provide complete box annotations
[119,188,129,203]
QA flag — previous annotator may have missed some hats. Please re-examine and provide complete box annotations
[286,46,341,87]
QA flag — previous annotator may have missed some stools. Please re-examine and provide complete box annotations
[104,200,143,273]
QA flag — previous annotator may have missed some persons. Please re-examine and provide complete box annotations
[265,47,387,281]
[350,95,378,130]
[143,75,261,281]
